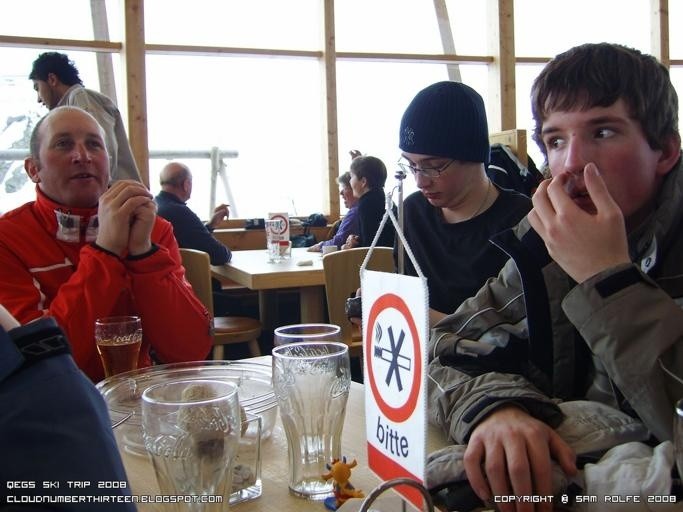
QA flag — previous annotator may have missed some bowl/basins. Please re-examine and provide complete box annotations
[98,361,279,454]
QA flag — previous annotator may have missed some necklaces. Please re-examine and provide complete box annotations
[470,176,491,218]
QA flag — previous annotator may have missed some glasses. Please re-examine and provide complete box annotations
[397,156,456,178]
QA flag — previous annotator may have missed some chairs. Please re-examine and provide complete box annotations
[313,247,399,381]
[178,247,262,362]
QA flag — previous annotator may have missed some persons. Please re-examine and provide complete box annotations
[309,150,393,250]
[0,304,141,512]
[29,51,142,190]
[326,170,361,239]
[321,456,365,511]
[427,44,683,512]
[0,104,214,382]
[350,80,537,331]
[149,161,232,317]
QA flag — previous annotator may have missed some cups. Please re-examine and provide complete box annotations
[94,317,140,408]
[264,219,280,264]
[279,240,291,260]
[229,413,263,505]
[273,324,337,342]
[270,342,350,498]
[144,382,237,511]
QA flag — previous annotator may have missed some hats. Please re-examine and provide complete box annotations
[396,80,494,164]
